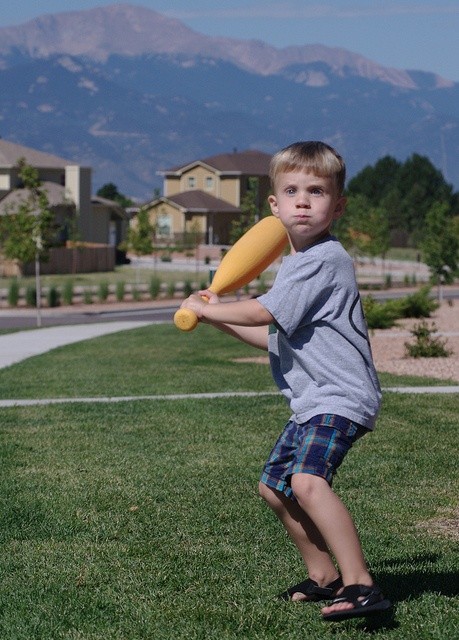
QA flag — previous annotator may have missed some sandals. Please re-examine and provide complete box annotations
[277,575,344,602]
[322,584,394,622]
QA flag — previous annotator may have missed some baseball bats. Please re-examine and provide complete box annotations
[175,216,289,329]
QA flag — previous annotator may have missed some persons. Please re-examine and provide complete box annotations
[180,142,392,621]
